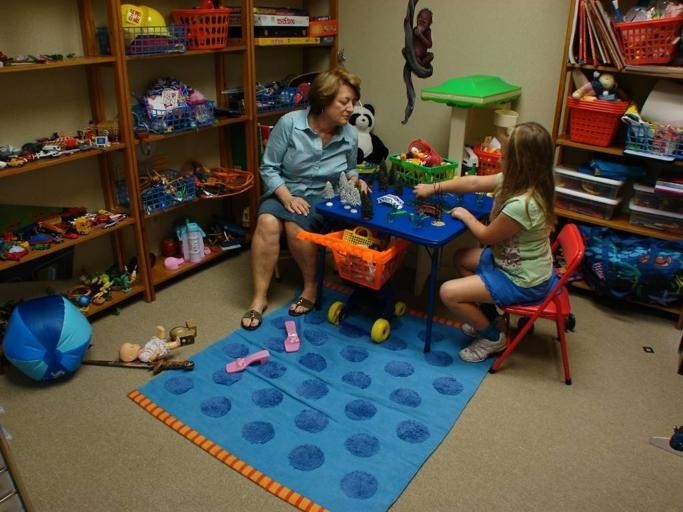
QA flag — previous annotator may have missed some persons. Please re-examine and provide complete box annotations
[413,122,554,362]
[241,68,372,330]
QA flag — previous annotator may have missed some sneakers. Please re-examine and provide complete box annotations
[458,332,506,363]
[461,323,477,338]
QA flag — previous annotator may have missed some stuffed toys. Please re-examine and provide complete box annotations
[348,100,389,165]
[572,71,617,99]
[401,0,433,125]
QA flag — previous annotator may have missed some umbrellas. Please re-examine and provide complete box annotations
[222,81,276,94]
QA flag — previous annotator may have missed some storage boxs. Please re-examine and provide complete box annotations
[633,183,682,214]
[627,196,682,235]
[551,164,623,199]
[552,186,623,221]
[227,16,309,38]
[568,92,626,148]
[611,15,675,64]
[307,21,338,38]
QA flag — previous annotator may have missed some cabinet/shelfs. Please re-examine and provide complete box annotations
[0,1,339,353]
[553,0,683,329]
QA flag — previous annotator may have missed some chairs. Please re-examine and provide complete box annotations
[489,224,585,385]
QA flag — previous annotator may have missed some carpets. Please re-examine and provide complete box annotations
[125,277,496,511]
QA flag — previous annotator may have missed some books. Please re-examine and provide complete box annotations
[577,1,626,70]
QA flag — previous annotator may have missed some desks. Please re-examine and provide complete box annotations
[316,182,495,353]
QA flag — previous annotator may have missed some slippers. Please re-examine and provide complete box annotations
[225,350,270,374]
[283,321,300,353]
[288,297,315,317]
[241,305,269,330]
